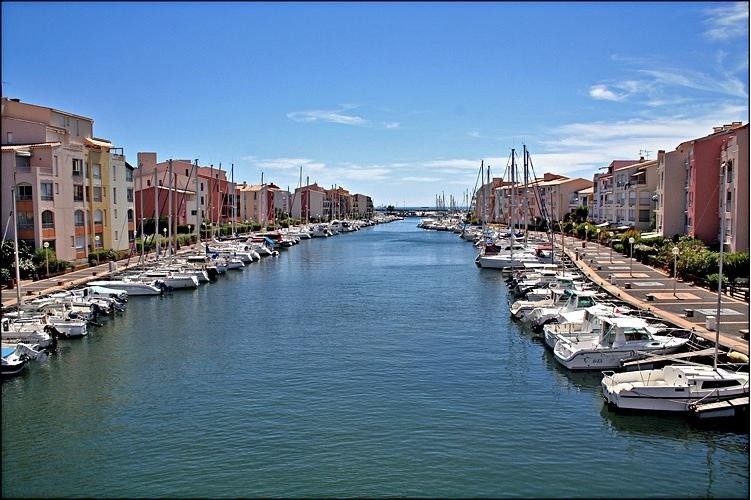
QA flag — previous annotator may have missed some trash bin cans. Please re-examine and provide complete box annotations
[705,315,716,331]
[8,279,14,289]
[611,275,616,285]
[34,274,39,281]
[109,261,117,271]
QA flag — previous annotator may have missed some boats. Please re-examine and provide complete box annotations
[405,210,416,217]
